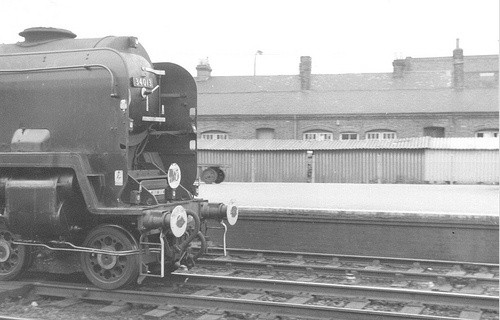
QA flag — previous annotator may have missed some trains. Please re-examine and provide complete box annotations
[0,25,237,290]
